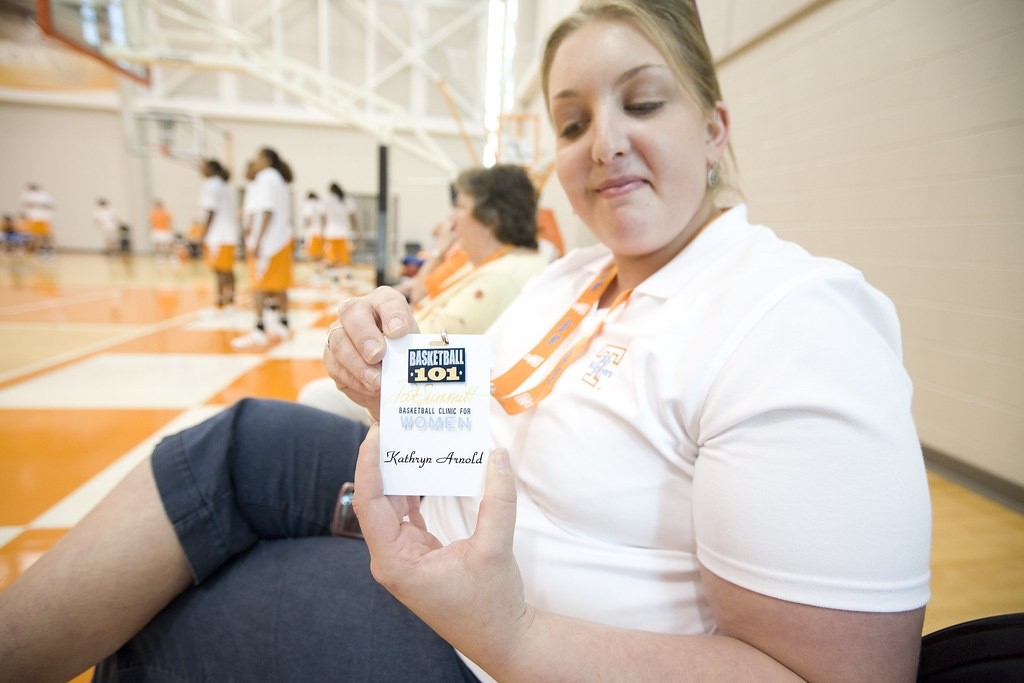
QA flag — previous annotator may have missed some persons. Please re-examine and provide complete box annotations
[297,163,549,427]
[18,183,54,254]
[149,148,363,348]
[0,0,932,683]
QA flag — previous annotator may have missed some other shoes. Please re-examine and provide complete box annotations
[202,304,236,319]
[265,319,289,335]
[233,329,267,347]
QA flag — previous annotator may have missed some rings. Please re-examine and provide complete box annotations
[326,326,344,351]
[399,515,410,525]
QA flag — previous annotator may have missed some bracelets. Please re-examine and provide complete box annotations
[431,249,445,262]
